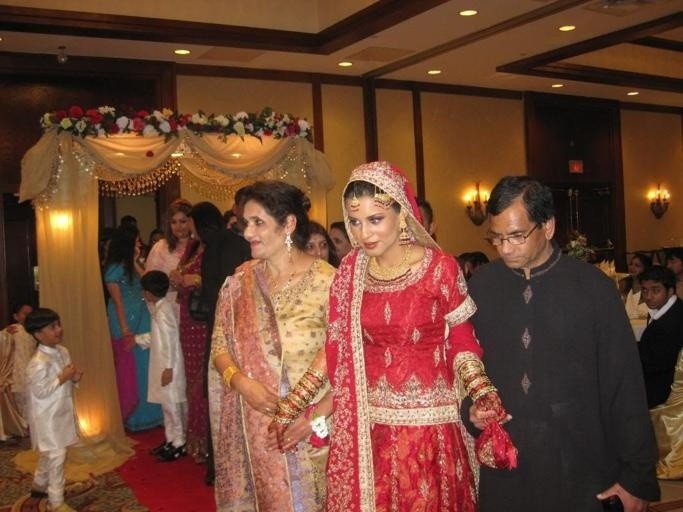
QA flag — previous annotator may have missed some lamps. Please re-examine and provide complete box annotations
[462,179,488,226]
[646,182,672,219]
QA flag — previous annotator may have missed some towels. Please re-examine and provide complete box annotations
[625,289,642,317]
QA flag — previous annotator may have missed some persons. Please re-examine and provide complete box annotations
[0,299,39,444]
[23,307,84,512]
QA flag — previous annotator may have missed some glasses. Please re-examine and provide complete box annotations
[483,224,541,249]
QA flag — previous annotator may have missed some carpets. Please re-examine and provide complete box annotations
[0,423,151,512]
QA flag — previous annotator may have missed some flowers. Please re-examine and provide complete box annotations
[565,231,595,259]
[310,407,329,440]
[38,102,315,144]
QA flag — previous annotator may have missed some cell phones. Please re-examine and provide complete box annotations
[602,495,624,512]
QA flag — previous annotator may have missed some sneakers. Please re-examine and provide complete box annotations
[150,442,173,456]
[30,480,68,498]
[160,442,189,462]
[44,500,76,512]
[0,433,23,448]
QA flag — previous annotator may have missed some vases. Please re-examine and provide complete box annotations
[578,255,591,263]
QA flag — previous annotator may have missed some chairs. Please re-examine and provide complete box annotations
[1,332,27,439]
[649,352,683,482]
